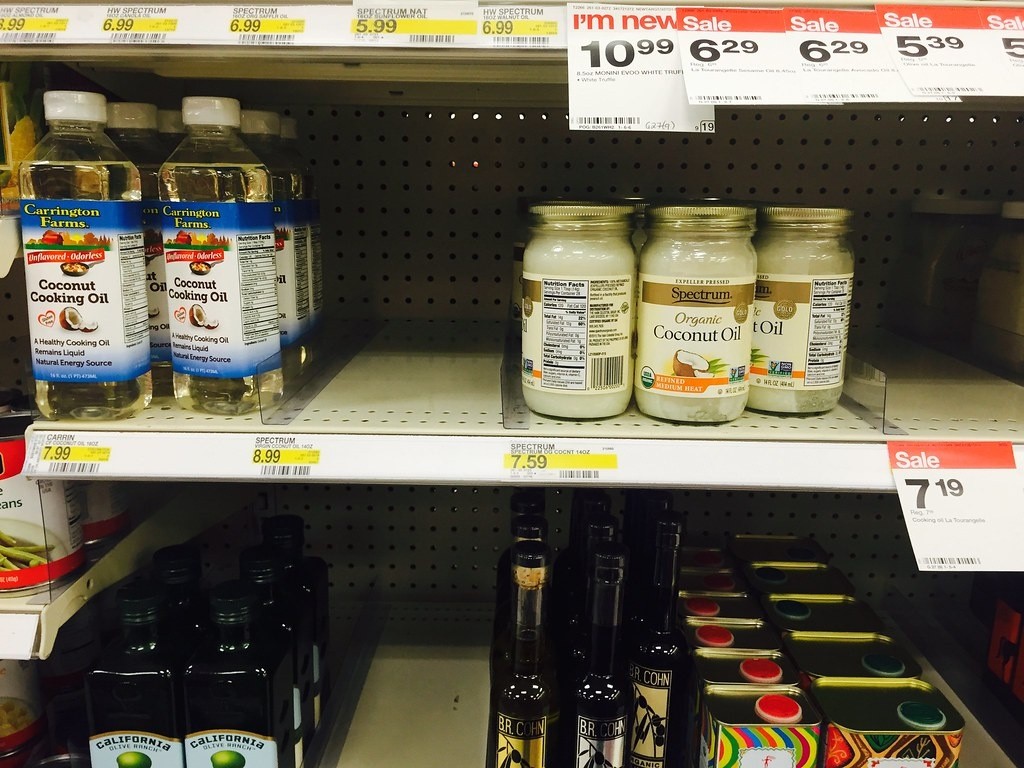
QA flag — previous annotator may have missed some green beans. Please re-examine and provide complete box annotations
[0,530,55,572]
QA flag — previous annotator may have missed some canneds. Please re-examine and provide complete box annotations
[523,204,853,423]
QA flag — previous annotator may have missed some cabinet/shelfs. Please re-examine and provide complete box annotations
[1,0,1024,767]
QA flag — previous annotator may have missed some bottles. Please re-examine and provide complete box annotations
[78,514,329,768]
[15,89,185,420]
[484,485,694,768]
[157,96,322,413]
